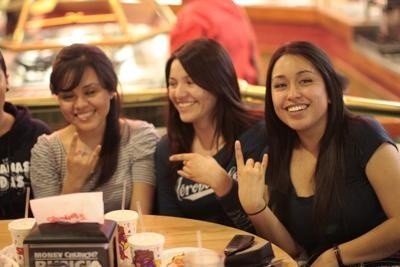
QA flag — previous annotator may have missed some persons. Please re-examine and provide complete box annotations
[167,0,258,86]
[30,43,160,216]
[234,41,400,267]
[153,39,268,235]
[0,49,53,219]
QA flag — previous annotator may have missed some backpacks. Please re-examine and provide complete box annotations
[223,234,276,267]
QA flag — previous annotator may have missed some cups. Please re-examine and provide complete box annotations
[127,232,164,267]
[8,218,36,267]
[104,210,138,267]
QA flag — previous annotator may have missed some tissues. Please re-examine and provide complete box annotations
[21,191,121,267]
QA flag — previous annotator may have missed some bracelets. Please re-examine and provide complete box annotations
[246,206,267,216]
[334,244,344,267]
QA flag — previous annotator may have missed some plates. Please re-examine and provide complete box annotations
[0,243,13,267]
[158,246,221,267]
